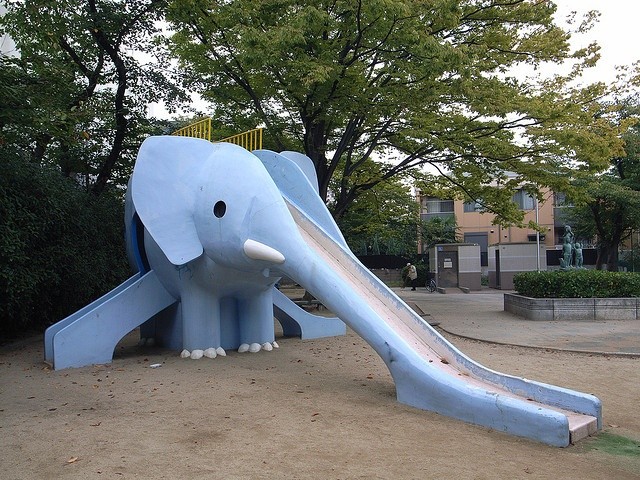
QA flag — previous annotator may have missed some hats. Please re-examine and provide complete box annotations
[407,262,410,266]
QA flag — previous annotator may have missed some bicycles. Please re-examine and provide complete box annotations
[425,278,437,293]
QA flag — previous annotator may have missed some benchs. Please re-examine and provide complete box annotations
[311,300,322,312]
[293,301,309,307]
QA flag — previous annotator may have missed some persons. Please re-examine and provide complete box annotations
[406,263,418,291]
[564,224,574,264]
[574,243,584,268]
[563,236,572,267]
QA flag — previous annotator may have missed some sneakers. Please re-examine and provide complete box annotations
[411,288,416,291]
